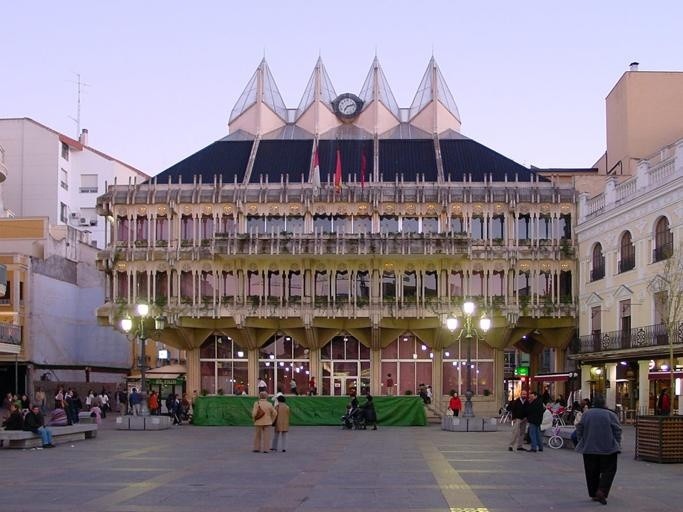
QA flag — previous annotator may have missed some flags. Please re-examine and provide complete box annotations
[359,146,367,192]
[333,149,342,194]
[308,141,322,197]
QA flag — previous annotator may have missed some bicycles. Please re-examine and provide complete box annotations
[547,420,565,449]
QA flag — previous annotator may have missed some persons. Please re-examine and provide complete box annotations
[575,396,622,505]
[270,395,290,452]
[526,392,546,452]
[252,391,277,453]
[616,387,671,418]
[236,373,463,431]
[509,391,528,451]
[507,390,590,448]
[1,384,198,449]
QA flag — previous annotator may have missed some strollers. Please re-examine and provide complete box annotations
[337,407,363,429]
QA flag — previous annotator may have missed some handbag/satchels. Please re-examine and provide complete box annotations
[255,401,265,420]
[271,405,279,426]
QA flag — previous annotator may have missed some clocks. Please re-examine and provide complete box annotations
[331,93,363,118]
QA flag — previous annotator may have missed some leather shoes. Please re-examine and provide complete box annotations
[509,447,543,453]
[594,494,607,505]
[43,444,56,448]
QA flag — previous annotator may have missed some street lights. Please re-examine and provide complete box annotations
[118,300,167,416]
[445,300,490,418]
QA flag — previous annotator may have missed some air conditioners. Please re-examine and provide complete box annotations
[79,218,90,226]
[72,213,79,218]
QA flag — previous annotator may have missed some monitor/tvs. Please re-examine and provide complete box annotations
[513,367,530,377]
[159,349,168,360]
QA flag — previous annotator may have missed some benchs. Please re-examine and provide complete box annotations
[544,425,577,450]
[0,424,97,449]
